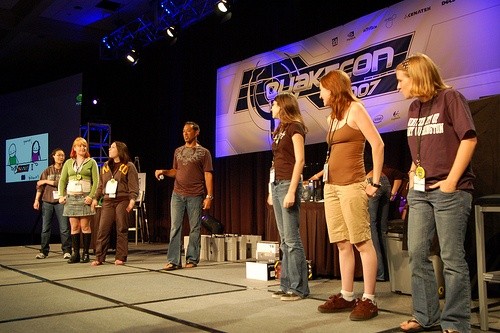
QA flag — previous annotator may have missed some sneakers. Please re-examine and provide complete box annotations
[272,290,286,298]
[350,297,378,321]
[64,253,71,258]
[36,252,46,259]
[281,292,302,300]
[317,293,360,313]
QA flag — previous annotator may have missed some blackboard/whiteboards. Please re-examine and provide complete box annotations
[135,173,146,201]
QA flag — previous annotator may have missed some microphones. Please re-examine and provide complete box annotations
[159,174,164,179]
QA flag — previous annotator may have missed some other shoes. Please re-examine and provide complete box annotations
[185,262,197,268]
[115,260,124,265]
[91,260,103,266]
[163,262,182,270]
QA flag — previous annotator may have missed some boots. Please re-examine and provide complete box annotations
[67,233,80,263]
[80,233,92,263]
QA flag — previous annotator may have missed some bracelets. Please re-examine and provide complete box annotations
[206,195,213,200]
[370,182,381,188]
[408,170,416,175]
[313,174,320,180]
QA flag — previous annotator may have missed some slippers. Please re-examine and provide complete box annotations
[399,318,441,333]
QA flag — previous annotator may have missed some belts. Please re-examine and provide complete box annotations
[273,182,283,186]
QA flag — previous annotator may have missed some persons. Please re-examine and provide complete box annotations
[58,137,99,263]
[155,122,213,271]
[91,142,139,266]
[267,94,310,301]
[304,142,391,282]
[395,55,477,333]
[317,70,384,321]
[33,149,73,259]
